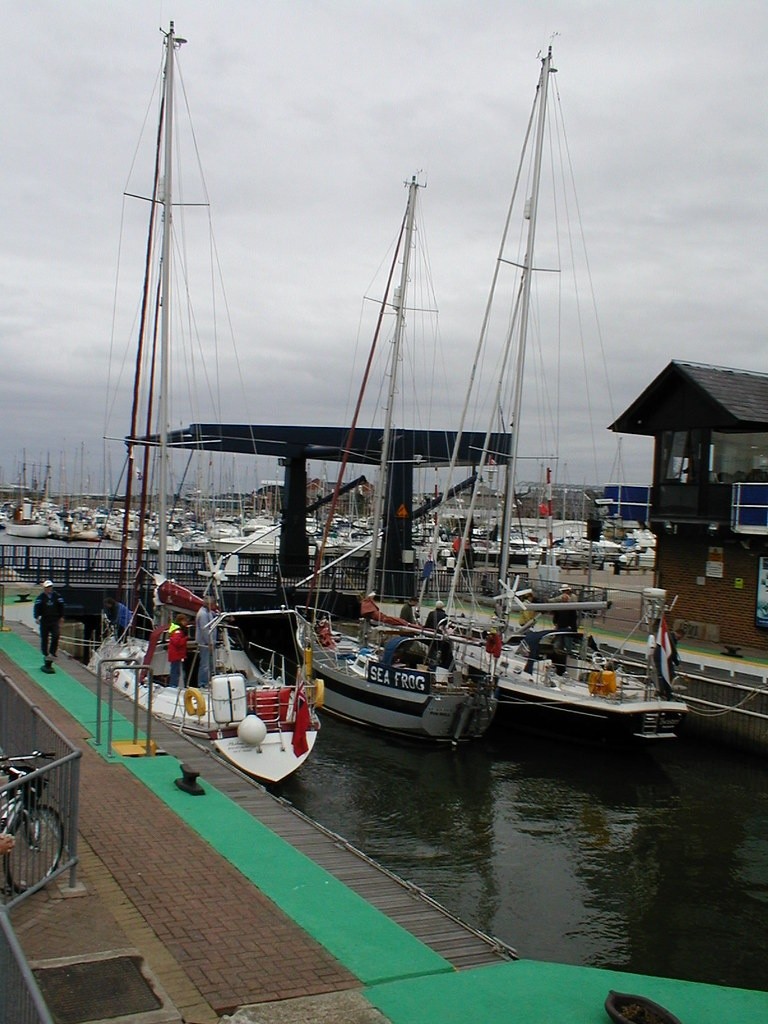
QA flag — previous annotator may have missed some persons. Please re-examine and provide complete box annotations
[103,597,131,644]
[371,595,448,669]
[652,627,685,696]
[191,595,220,687]
[34,580,66,657]
[549,584,580,631]
[168,616,195,686]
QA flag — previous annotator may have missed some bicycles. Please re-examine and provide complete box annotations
[0,750,65,894]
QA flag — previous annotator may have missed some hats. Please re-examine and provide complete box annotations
[558,584,571,592]
[44,580,54,587]
[435,601,446,608]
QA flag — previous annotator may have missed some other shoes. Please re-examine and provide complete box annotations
[49,649,58,657]
[45,654,51,660]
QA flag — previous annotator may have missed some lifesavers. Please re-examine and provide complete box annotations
[486,632,502,658]
[588,672,606,696]
[183,690,206,717]
[314,678,327,708]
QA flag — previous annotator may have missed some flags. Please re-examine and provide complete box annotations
[292,671,314,758]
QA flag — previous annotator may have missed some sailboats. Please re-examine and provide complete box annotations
[0,14,688,783]
